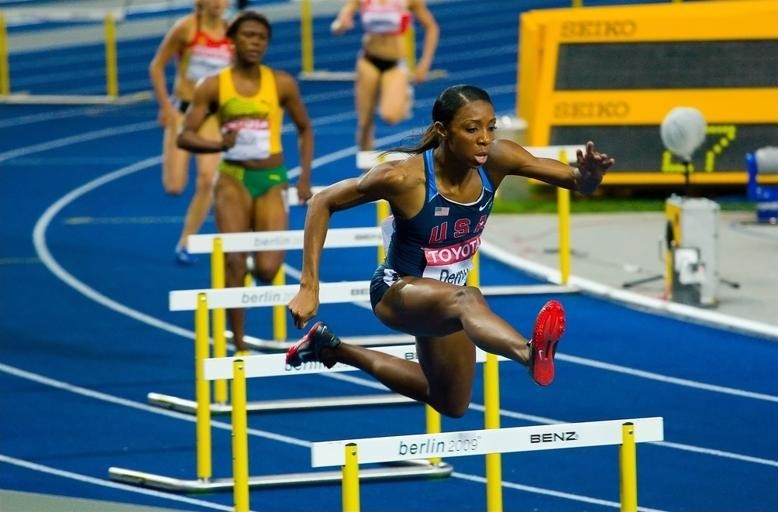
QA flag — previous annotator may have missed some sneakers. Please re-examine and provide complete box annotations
[243,269,255,288]
[232,348,251,358]
[530,299,567,387]
[284,320,341,369]
[175,246,200,267]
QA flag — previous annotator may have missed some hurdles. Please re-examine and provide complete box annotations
[108,279,453,493]
[147,226,419,416]
[290,0,447,83]
[311,416,664,512]
[203,344,513,511]
[356,145,587,295]
[1,5,155,105]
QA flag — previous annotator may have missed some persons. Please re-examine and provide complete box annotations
[145,0,237,266]
[283,82,614,419]
[173,8,315,356]
[328,0,439,149]
